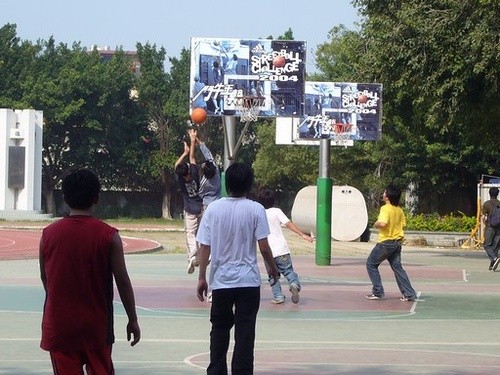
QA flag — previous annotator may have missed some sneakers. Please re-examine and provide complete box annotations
[399,296,417,301]
[289,284,299,304]
[270,299,284,304]
[365,294,383,300]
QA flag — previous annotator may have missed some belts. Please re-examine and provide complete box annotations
[383,239,402,243]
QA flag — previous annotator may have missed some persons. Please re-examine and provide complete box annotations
[173,129,223,273]
[194,161,277,375]
[38,168,141,375]
[300,96,325,138]
[365,185,417,301]
[481,187,500,271]
[194,53,239,112]
[252,185,316,305]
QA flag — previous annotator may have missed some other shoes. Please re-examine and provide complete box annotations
[187,256,199,274]
[489,257,500,271]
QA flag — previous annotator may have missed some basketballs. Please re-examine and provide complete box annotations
[191,108,207,124]
[274,55,286,67]
[359,95,367,103]
[214,40,220,46]
